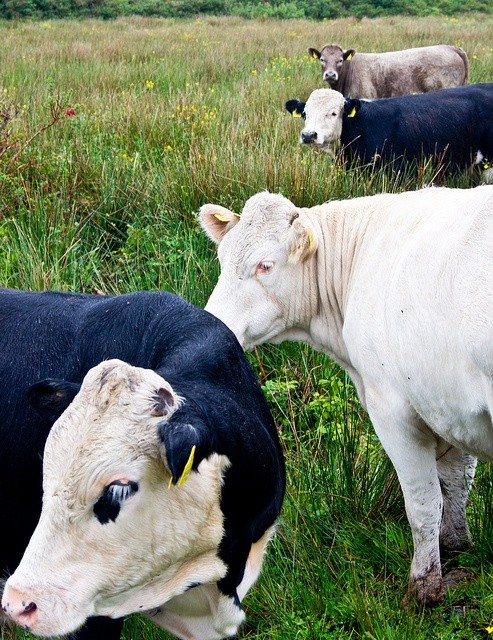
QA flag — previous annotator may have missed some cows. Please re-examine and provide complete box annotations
[199,184,492,611]
[0,287,287,639]
[284,84,493,190]
[308,45,469,99]
